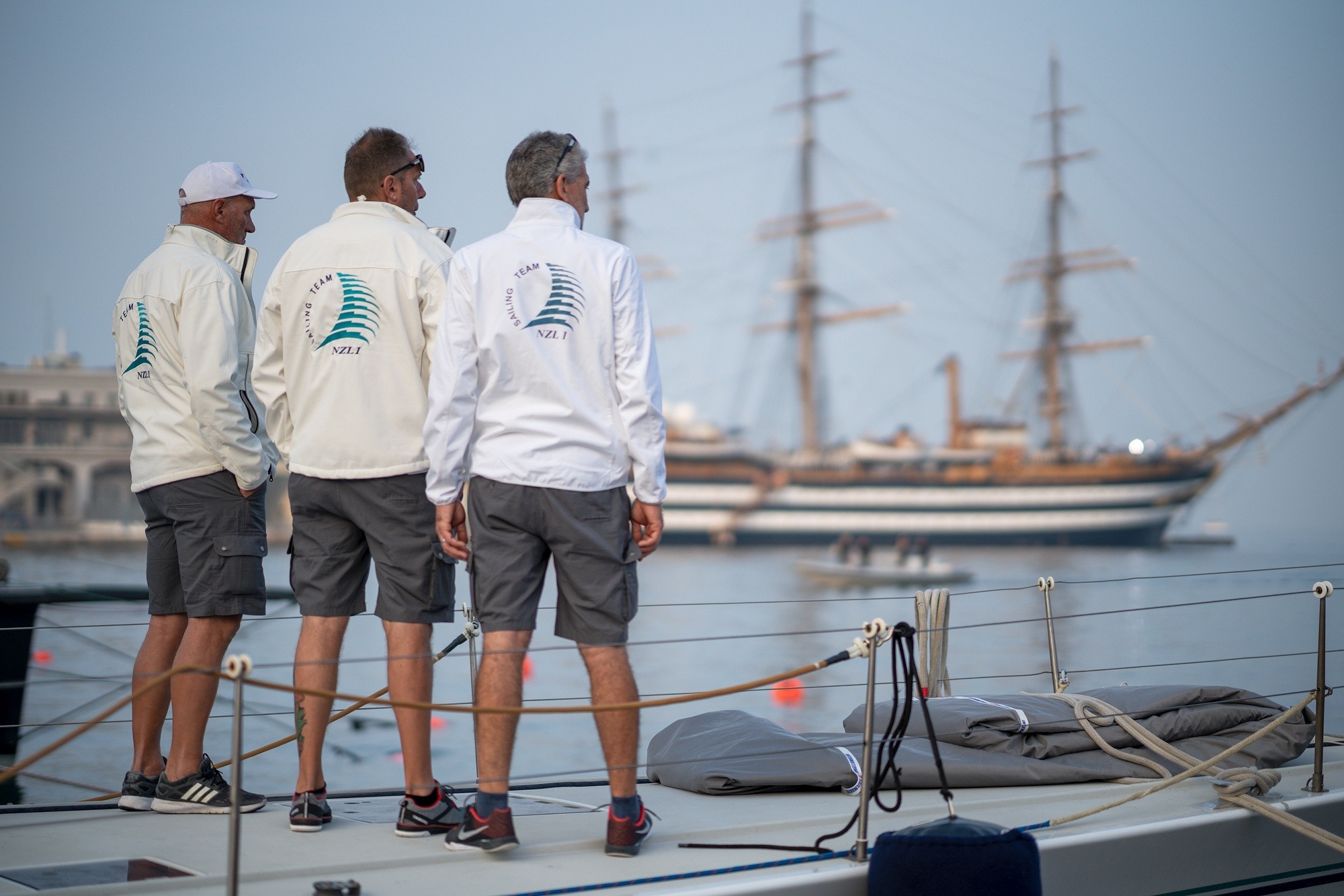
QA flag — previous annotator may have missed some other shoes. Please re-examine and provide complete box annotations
[0,775,24,806]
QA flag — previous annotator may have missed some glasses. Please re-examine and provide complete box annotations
[556,132,579,171]
[379,154,426,187]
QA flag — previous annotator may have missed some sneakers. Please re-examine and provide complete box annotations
[151,752,267,814]
[118,754,169,810]
[289,781,333,832]
[442,794,519,852]
[394,779,466,838]
[593,802,662,857]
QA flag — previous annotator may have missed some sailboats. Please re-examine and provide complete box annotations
[598,7,1344,551]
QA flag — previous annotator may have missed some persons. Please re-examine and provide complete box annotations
[113,160,279,814]
[250,128,465,839]
[424,131,668,858]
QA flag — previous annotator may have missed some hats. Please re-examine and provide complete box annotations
[177,161,278,207]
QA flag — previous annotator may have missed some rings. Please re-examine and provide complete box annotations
[440,539,446,544]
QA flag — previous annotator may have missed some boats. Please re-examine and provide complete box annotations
[0,562,1344,896]
[795,557,973,589]
